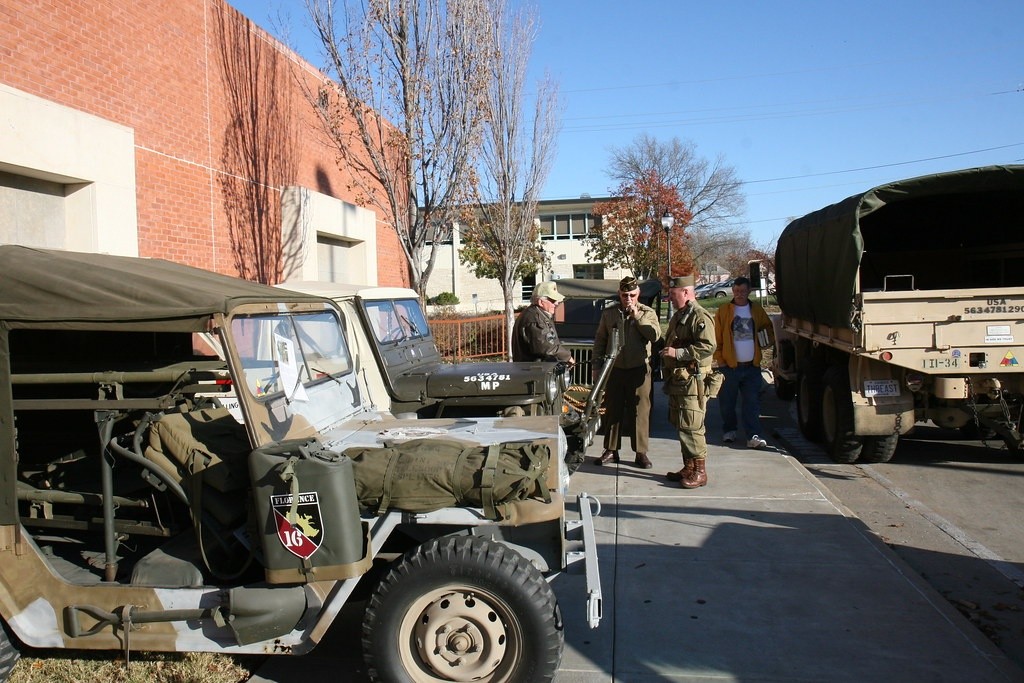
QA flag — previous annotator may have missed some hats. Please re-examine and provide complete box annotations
[532,281,565,301]
[620,276,638,292]
[666,272,695,288]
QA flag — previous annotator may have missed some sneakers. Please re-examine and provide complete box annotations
[723,430,737,442]
[746,435,767,447]
[634,453,652,468]
[593,449,620,466]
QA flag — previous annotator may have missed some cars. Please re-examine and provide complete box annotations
[700,282,727,299]
[694,284,714,298]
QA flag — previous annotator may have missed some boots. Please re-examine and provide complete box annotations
[667,456,694,481]
[680,458,708,489]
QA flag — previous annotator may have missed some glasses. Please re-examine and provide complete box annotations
[621,294,636,298]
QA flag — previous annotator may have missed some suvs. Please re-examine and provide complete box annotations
[549,279,661,414]
[0,245,604,683]
[269,281,603,472]
[709,276,744,299]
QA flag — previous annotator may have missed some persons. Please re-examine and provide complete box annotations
[512,280,575,363]
[713,278,777,446]
[659,275,718,489]
[592,276,661,469]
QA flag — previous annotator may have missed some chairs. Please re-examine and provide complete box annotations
[144,402,252,524]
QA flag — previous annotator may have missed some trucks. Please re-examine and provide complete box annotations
[746,165,1024,462]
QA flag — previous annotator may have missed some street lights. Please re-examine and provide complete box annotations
[661,206,676,319]
